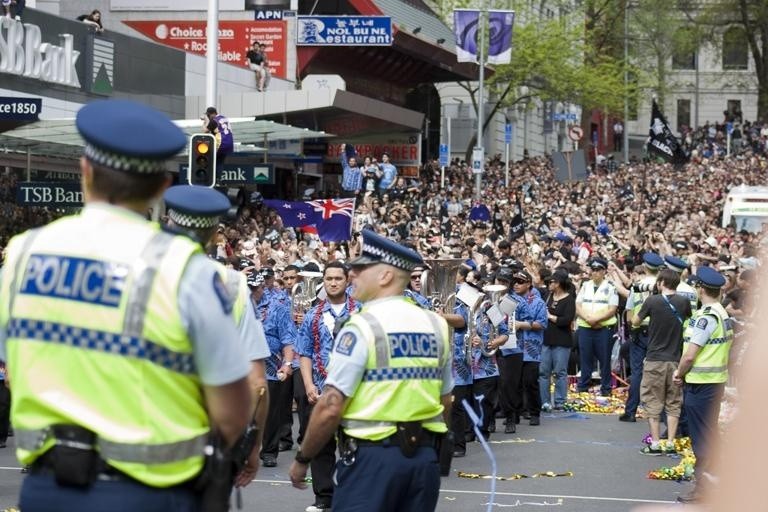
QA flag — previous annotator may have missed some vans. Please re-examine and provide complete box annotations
[723,188,768,234]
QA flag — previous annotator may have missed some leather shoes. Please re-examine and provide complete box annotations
[452,407,543,457]
[677,490,706,504]
[263,455,277,467]
[278,440,293,451]
[619,414,636,422]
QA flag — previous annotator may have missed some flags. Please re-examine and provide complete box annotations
[646,100,689,162]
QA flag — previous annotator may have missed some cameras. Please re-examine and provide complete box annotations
[633,283,661,295]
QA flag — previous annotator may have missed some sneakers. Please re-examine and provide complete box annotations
[305,502,331,512]
[665,446,677,457]
[638,444,662,456]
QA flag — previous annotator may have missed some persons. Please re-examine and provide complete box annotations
[295,261,361,511]
[183,104,768,467]
[672,267,732,504]
[0,97,251,511]
[0,171,86,267]
[261,44,274,89]
[246,40,265,93]
[160,184,271,489]
[11,0,26,20]
[290,228,455,511]
[79,10,104,28]
[2,0,11,17]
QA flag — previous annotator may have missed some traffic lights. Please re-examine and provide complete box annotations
[189,136,217,188]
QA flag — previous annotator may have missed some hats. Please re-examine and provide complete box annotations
[480,222,609,283]
[696,266,726,290]
[206,108,216,115]
[702,236,718,249]
[241,258,274,287]
[350,228,424,271]
[664,256,688,273]
[163,185,231,229]
[76,98,187,175]
[643,253,664,271]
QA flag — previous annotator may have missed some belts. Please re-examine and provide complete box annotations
[356,431,441,446]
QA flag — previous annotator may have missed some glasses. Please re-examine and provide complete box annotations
[410,275,421,279]
[548,280,556,284]
[512,278,524,284]
[284,276,296,280]
